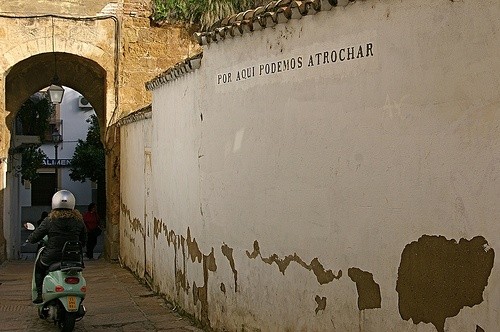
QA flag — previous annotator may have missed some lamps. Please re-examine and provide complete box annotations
[48,55,64,105]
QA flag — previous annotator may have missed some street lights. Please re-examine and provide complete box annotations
[52,129,63,190]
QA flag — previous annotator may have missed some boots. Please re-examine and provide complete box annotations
[33,287,43,303]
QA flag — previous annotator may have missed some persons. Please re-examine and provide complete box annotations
[26,190,102,304]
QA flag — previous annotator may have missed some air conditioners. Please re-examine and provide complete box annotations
[79,96,92,108]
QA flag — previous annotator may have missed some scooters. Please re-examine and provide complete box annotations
[24,222,88,332]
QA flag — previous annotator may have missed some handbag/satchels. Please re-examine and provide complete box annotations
[92,227,102,236]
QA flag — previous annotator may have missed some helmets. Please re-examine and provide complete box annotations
[52,190,75,210]
